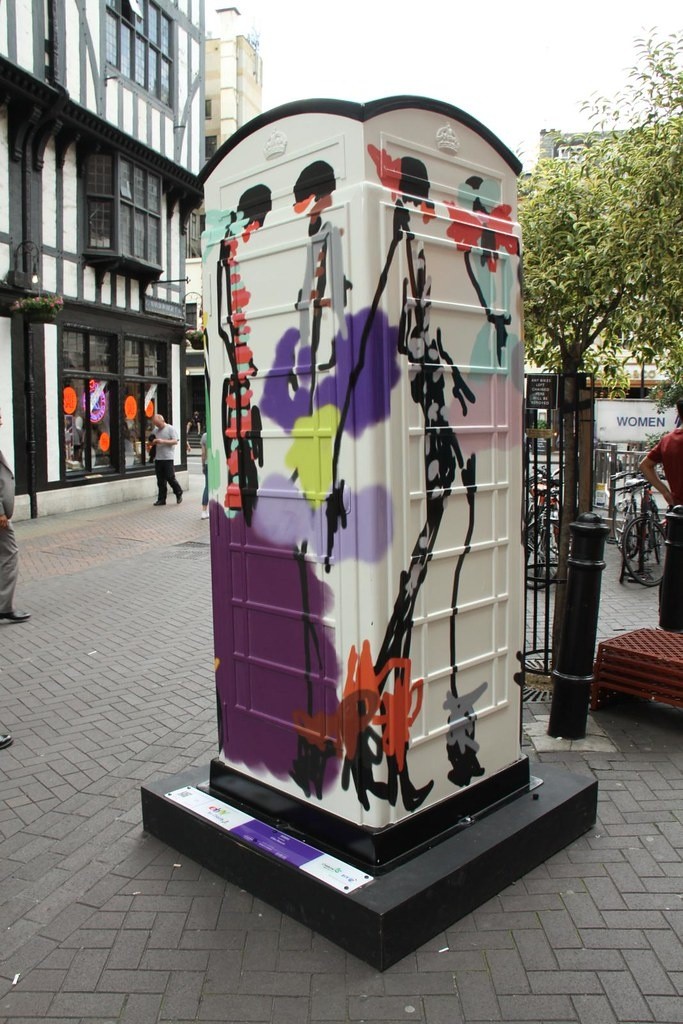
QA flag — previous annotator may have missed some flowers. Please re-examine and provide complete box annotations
[186,330,203,342]
[10,295,64,313]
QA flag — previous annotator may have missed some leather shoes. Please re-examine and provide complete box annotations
[177,494,182,503]
[153,500,166,505]
[0,608,31,620]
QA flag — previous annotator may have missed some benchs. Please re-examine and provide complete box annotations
[590,629,683,711]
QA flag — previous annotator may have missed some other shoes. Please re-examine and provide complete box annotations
[202,510,209,519]
[0,734,13,749]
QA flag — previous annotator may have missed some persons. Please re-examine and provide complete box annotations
[62,414,89,470]
[150,413,183,505]
[638,397,683,635]
[199,426,210,519]
[187,410,203,437]
[0,406,31,622]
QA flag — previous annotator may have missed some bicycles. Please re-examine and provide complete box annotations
[522,464,565,590]
[609,470,668,587]
